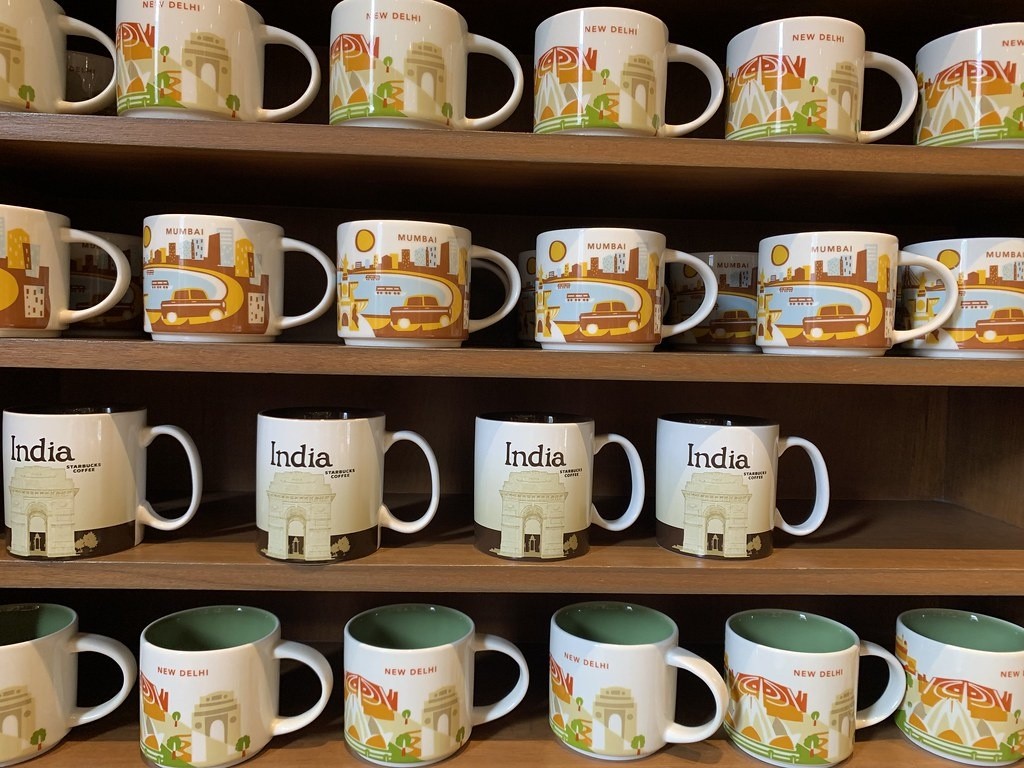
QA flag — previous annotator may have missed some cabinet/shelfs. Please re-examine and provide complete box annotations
[0,110,1024,768]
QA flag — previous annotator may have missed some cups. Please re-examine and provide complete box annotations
[474,412,646,561]
[755,231,959,355]
[532,6,724,137]
[723,609,907,767]
[255,408,441,561]
[117,0,320,123]
[900,236,1024,358]
[325,0,523,130]
[343,603,529,767]
[2,400,202,559]
[726,16,918,143]
[0,603,138,768]
[334,219,758,352]
[0,0,116,114]
[0,202,336,343]
[895,608,1024,766]
[139,605,333,768]
[655,416,829,560]
[915,21,1024,148]
[549,603,730,761]
